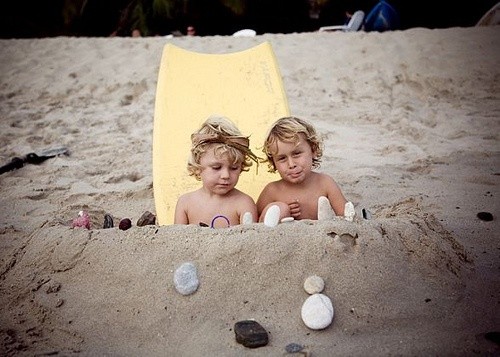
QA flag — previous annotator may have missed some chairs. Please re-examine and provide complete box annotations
[318,10,365,34]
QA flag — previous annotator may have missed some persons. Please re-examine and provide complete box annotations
[255,117,347,223]
[173,117,259,230]
